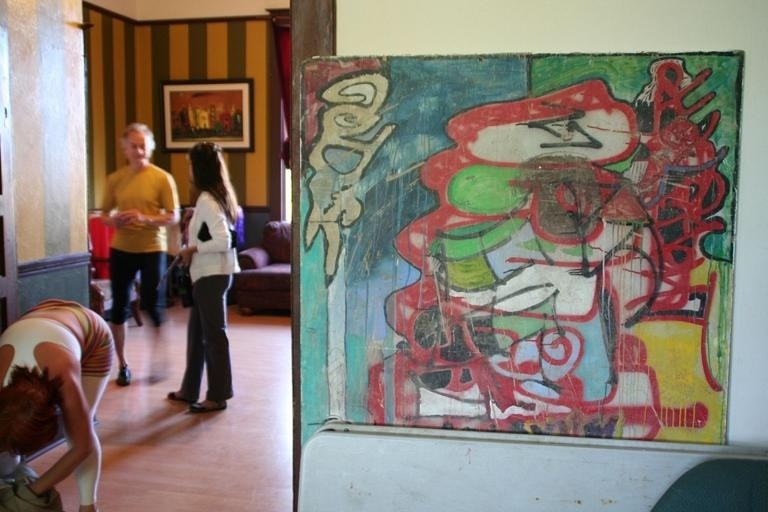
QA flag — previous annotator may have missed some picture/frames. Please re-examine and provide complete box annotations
[157,77,256,156]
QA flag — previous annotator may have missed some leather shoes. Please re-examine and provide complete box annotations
[117,367,131,386]
[168,392,197,404]
[189,400,228,412]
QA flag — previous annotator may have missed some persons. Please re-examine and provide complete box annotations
[100,123,181,386]
[0,297,115,512]
[167,141,242,413]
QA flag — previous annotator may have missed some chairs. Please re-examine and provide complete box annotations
[231,215,293,321]
[87,250,146,331]
